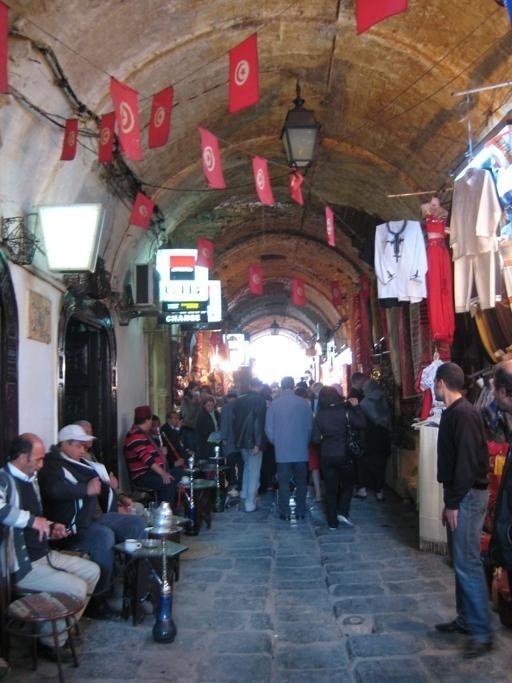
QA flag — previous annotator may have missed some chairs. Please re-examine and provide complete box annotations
[5,527,82,682]
[132,484,159,507]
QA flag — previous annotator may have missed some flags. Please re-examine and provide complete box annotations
[325,206,338,247]
[289,172,304,205]
[196,234,216,270]
[59,118,78,161]
[350,0,410,35]
[226,34,261,112]
[148,85,174,148]
[248,264,266,295]
[250,156,275,205]
[331,282,343,305]
[197,125,228,190]
[293,277,307,305]
[99,112,116,163]
[130,192,155,228]
[358,275,369,297]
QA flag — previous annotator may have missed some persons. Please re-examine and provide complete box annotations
[0,431,99,663]
[487,358,512,629]
[421,196,455,342]
[74,419,147,519]
[152,368,396,530]
[38,424,147,617]
[124,405,189,508]
[433,364,496,658]
[473,376,503,433]
[108,74,143,162]
[0,3,11,93]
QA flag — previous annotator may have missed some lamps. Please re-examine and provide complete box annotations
[281,79,322,168]
[2,201,105,275]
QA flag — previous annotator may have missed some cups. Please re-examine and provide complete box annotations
[125,537,142,553]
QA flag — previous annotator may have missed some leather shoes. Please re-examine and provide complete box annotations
[281,515,291,521]
[82,598,124,621]
[462,639,493,658]
[434,620,470,634]
[296,515,304,520]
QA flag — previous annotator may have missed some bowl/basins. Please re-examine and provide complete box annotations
[140,538,161,548]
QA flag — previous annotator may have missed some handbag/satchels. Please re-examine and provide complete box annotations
[344,400,361,465]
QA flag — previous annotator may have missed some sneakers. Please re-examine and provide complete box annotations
[353,491,368,498]
[376,488,383,501]
[311,497,323,503]
[338,511,354,530]
[37,635,82,663]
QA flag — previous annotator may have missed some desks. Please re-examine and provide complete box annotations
[114,512,192,627]
[177,458,230,536]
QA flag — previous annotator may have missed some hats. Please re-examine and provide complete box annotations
[58,424,97,443]
[135,406,151,420]
